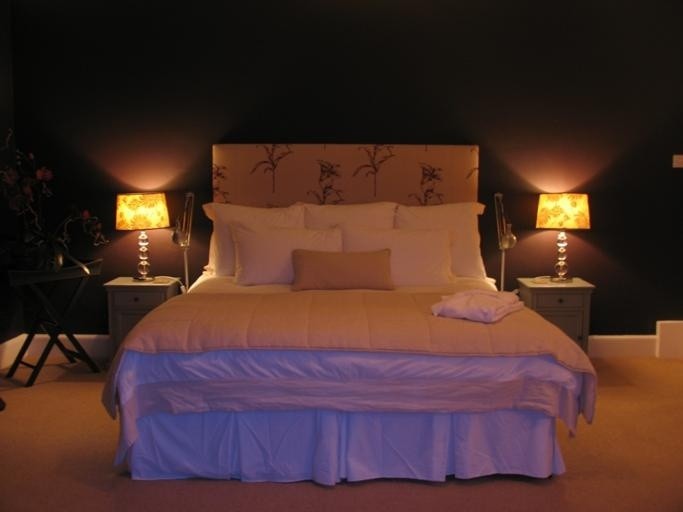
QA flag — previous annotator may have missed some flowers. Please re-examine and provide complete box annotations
[1,126,111,247]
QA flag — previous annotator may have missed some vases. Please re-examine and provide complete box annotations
[14,198,50,269]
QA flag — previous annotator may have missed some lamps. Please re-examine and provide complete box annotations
[535,193,590,283]
[115,193,170,281]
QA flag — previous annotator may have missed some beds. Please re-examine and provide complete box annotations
[101,143,596,488]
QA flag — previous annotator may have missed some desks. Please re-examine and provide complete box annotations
[0,256,103,387]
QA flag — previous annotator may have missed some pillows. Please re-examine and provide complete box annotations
[198,196,486,290]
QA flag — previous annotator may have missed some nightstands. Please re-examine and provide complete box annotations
[104,277,182,357]
[517,277,595,354]
